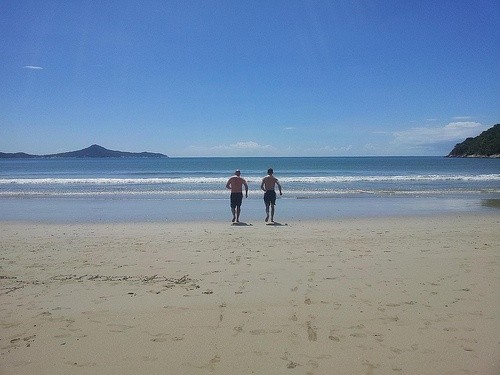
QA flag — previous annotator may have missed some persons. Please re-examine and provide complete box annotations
[225,169,248,221]
[261,168,282,222]
